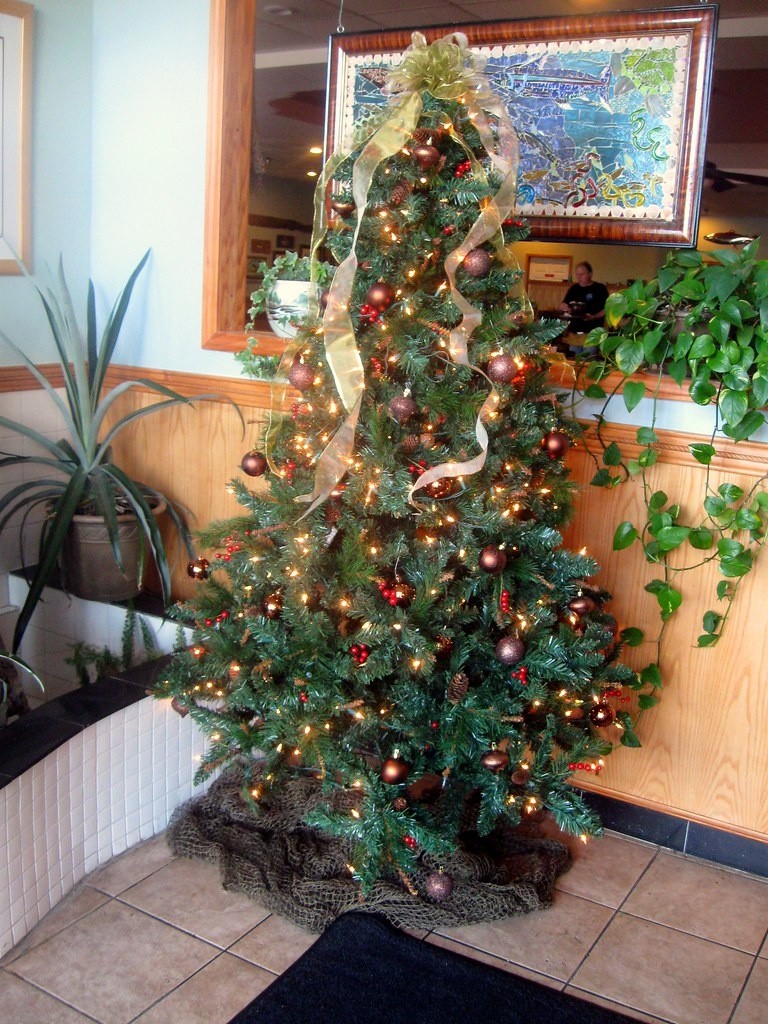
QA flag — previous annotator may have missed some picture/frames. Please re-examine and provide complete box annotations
[0,0,35,274]
[323,3,718,253]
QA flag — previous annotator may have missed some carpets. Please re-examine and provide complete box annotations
[228,911,648,1024]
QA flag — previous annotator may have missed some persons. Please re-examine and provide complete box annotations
[559,261,609,361]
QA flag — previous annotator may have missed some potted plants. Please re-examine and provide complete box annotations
[0,233,243,654]
[232,250,339,381]
[557,237,768,750]
